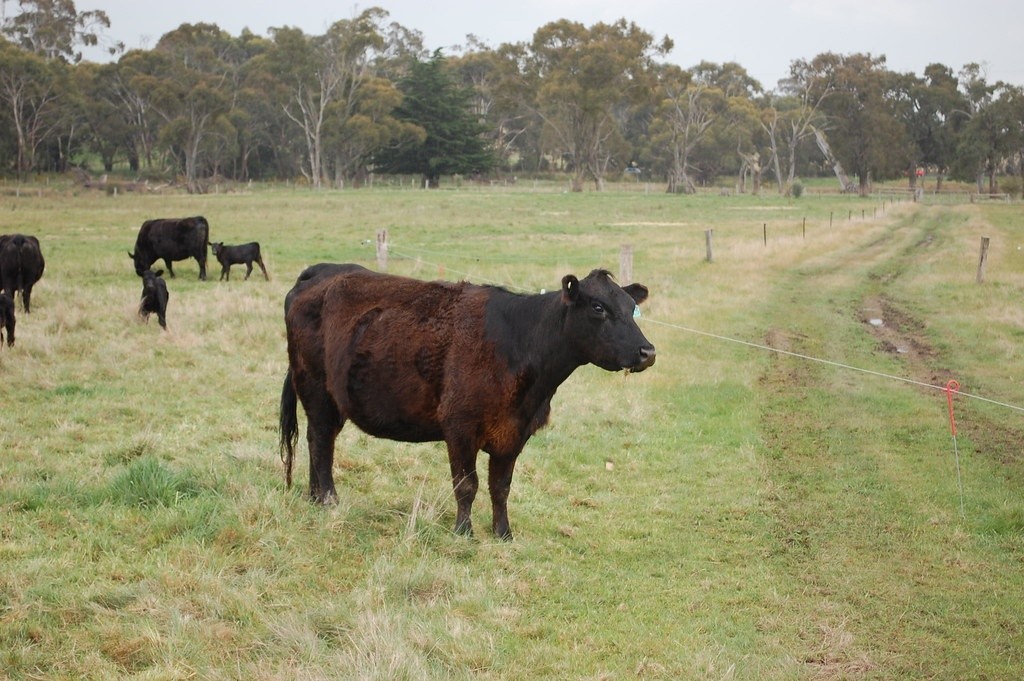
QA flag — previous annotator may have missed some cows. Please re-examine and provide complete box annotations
[128,215,209,282]
[0,234,45,351]
[279,263,657,541]
[136,267,169,331]
[208,241,269,282]
